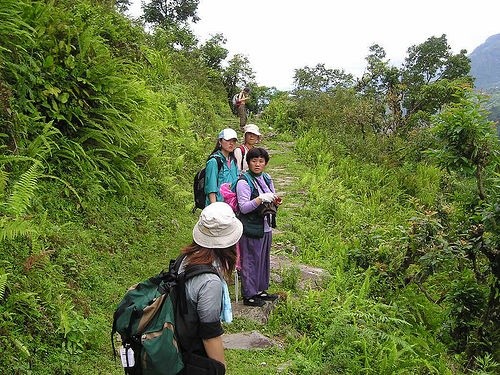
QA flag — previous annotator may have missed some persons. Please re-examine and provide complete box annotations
[232,148,282,308]
[204,128,242,207]
[164,201,243,375]
[233,124,261,179]
[235,88,250,127]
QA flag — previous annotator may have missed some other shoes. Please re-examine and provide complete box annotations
[243,295,265,306]
[257,290,277,301]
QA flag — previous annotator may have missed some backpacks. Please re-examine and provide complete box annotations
[194,152,237,210]
[111,253,223,375]
[222,172,271,217]
[233,92,246,107]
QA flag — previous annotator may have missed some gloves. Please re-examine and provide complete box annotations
[257,192,283,208]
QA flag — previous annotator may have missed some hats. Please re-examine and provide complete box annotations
[219,128,238,142]
[192,202,243,249]
[244,124,262,136]
[244,87,250,92]
